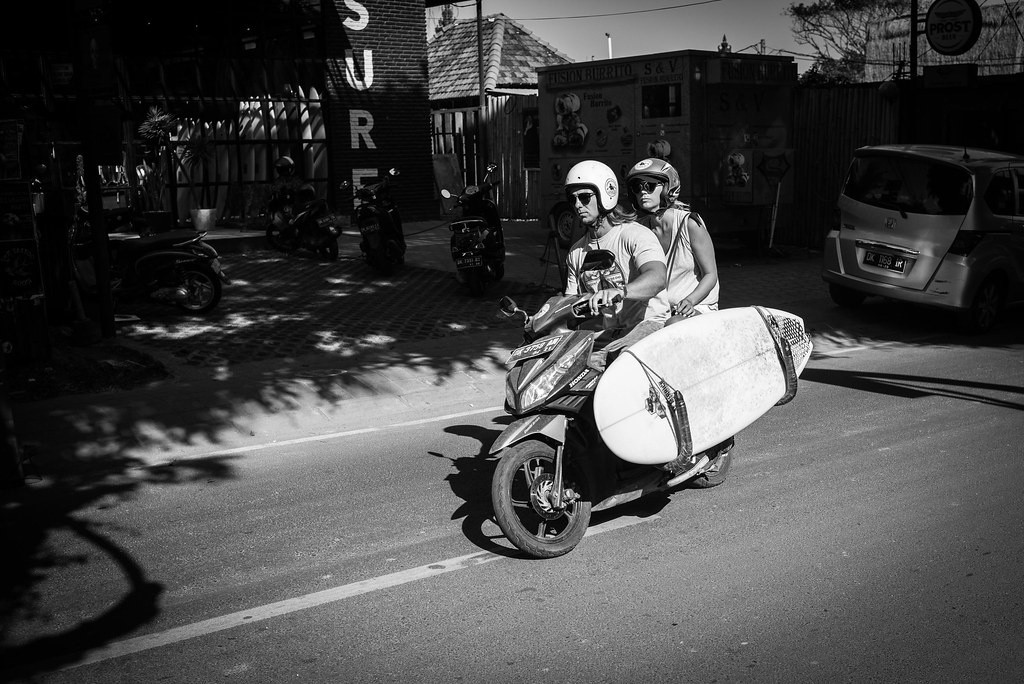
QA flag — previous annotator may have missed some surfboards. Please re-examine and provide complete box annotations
[592,304,815,465]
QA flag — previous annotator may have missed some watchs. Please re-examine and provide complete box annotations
[618,283,627,298]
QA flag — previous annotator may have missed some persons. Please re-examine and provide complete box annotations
[626,158,720,328]
[565,160,674,487]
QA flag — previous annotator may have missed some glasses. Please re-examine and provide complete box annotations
[567,192,597,207]
[628,181,664,194]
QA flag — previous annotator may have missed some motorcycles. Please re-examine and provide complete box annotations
[62,214,233,318]
[486,249,736,561]
[336,167,406,278]
[439,162,506,297]
[258,155,342,261]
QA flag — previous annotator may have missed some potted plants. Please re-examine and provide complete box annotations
[138,105,218,232]
[137,158,172,233]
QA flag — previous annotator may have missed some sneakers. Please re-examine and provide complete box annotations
[667,452,710,488]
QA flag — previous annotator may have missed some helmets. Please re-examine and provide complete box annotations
[624,158,681,215]
[562,159,619,216]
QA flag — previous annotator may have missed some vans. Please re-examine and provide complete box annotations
[818,141,1024,333]
